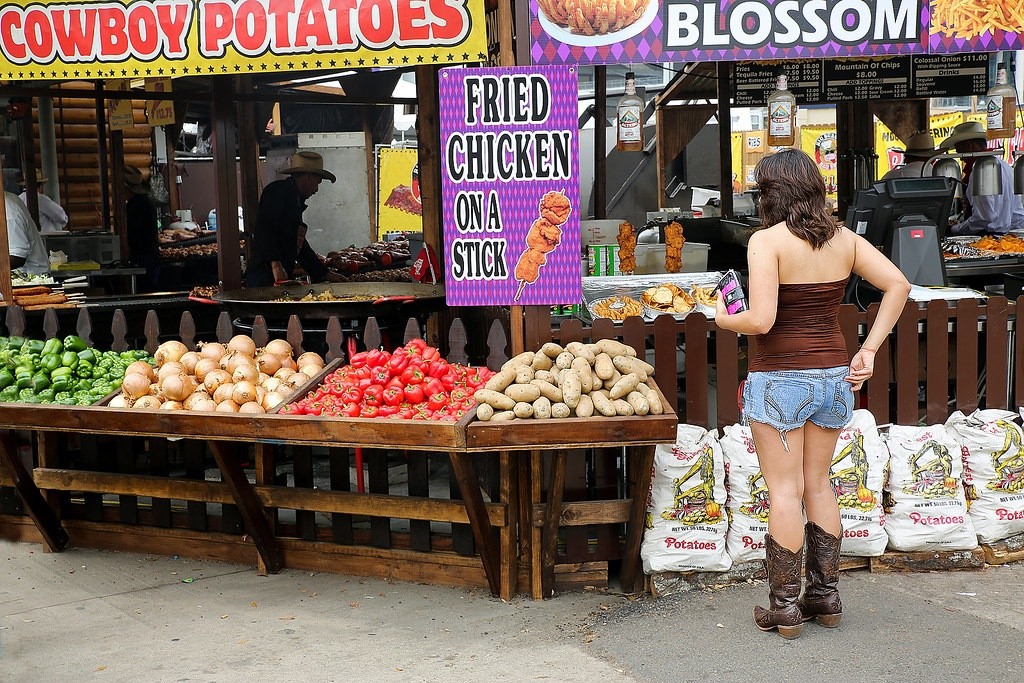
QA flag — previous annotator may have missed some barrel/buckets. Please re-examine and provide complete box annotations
[208,208,216,230]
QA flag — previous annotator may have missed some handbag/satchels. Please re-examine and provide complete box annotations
[146,155,170,205]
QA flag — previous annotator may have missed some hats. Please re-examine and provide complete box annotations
[940,122,988,149]
[120,164,148,194]
[280,151,336,184]
[17,168,48,185]
[892,130,949,157]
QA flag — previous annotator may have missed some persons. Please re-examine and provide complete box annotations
[940,121,1024,232]
[714,148,911,640]
[18,168,68,231]
[880,134,934,179]
[241,152,349,284]
[0,154,51,339]
[123,165,171,348]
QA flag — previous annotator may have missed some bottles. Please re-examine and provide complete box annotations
[767,74,796,146]
[986,62,1016,138]
[616,72,645,152]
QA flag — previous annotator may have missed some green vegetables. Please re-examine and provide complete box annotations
[10,270,51,282]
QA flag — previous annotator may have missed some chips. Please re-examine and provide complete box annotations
[969,235,1024,253]
[644,284,695,315]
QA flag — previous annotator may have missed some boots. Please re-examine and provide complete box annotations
[796,520,843,628]
[752,532,804,639]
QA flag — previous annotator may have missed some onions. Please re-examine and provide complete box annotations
[108,334,324,413]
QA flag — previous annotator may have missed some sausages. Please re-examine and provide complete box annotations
[11,286,77,310]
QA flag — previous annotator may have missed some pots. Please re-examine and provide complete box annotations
[187,279,447,319]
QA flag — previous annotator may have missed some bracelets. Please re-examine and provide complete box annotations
[861,347,877,354]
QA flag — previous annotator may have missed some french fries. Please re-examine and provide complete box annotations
[929,0,1024,40]
[692,284,717,307]
[593,295,643,320]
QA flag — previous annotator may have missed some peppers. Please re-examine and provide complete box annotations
[0,335,156,405]
[278,339,496,421]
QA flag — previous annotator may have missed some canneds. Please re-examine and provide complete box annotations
[549,304,583,316]
[588,244,624,276]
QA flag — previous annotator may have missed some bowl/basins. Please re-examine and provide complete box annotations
[538,0,658,48]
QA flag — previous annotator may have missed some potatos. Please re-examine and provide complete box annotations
[474,338,663,420]
